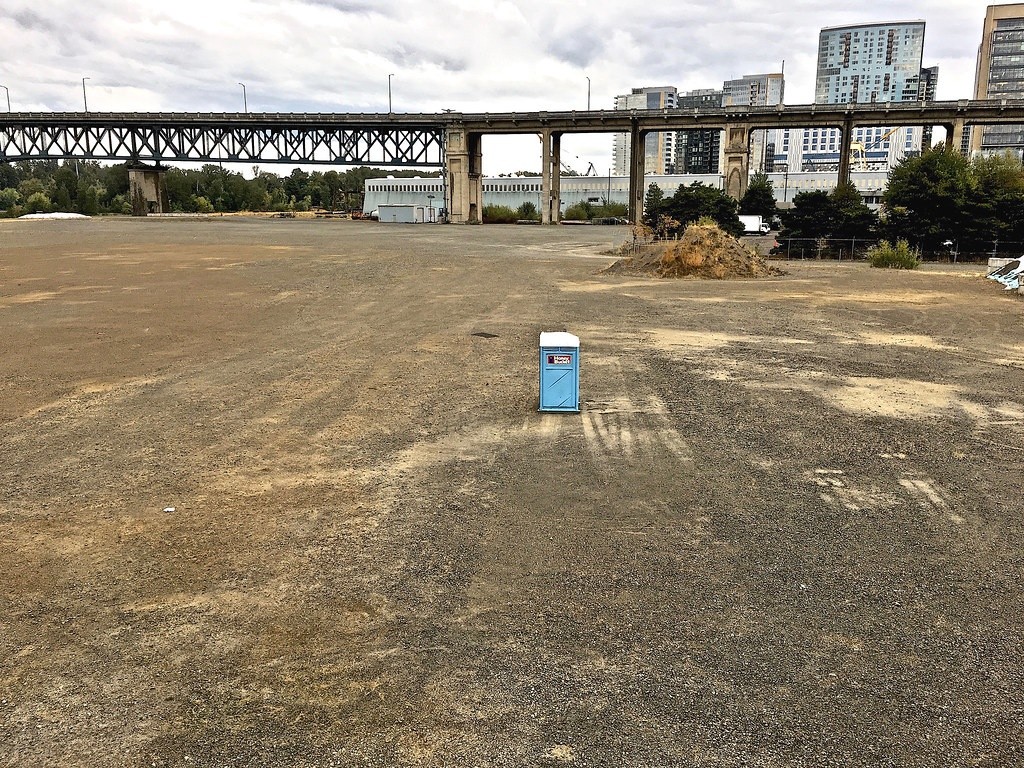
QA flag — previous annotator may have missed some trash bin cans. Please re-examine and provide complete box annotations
[537,332,581,412]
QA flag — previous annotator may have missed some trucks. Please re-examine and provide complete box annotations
[737,214,772,236]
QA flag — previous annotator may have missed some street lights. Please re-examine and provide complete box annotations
[586,77,590,111]
[0,84,11,112]
[389,74,395,113]
[237,83,248,113]
[428,195,435,223]
[718,174,727,191]
[82,77,91,117]
